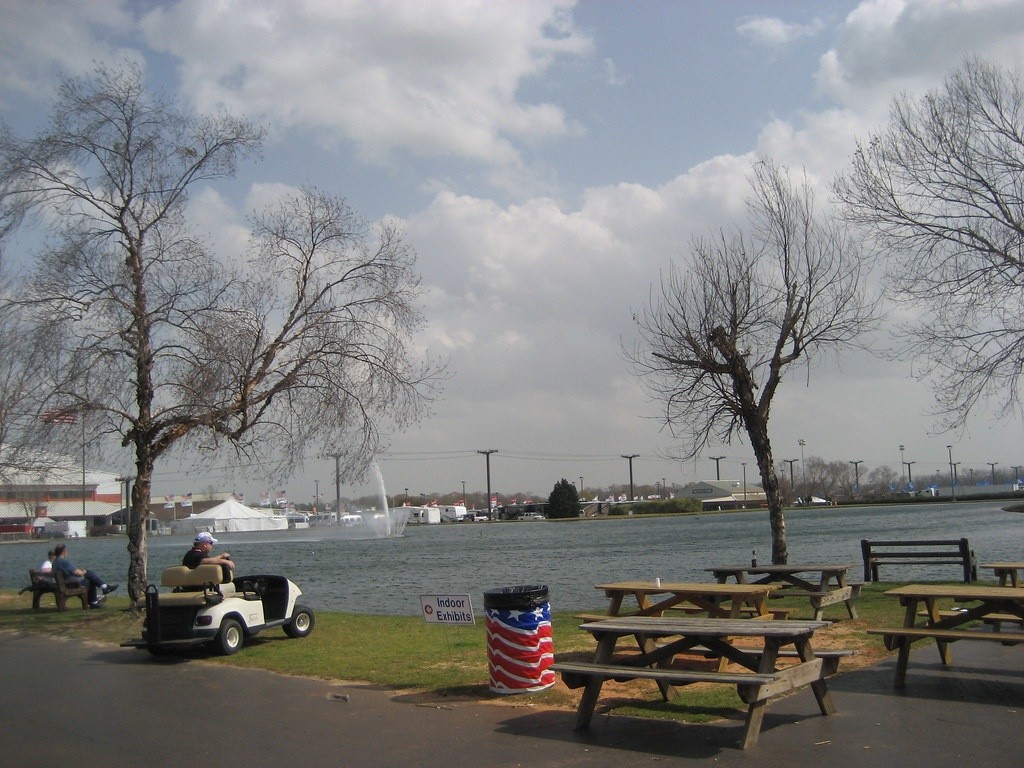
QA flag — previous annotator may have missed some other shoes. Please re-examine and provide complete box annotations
[88,601,101,608]
[102,584,119,594]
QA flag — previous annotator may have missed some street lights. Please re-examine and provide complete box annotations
[461,481,465,506]
[899,445,905,482]
[742,463,747,506]
[314,480,320,516]
[798,438,808,506]
[947,445,956,502]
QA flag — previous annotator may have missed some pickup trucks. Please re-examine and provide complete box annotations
[518,512,546,520]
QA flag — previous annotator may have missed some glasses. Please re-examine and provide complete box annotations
[207,541,213,545]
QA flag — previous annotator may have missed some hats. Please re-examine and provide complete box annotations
[193,532,219,543]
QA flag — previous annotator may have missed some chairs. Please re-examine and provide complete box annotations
[222,562,271,621]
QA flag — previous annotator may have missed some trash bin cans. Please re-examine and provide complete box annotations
[484,585,556,693]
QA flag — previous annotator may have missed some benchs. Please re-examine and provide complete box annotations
[856,535,980,582]
[547,658,780,688]
[919,607,1022,622]
[731,580,874,588]
[773,585,835,601]
[669,600,799,613]
[29,568,89,612]
[157,566,223,605]
[656,641,856,665]
[867,624,1024,645]
[570,609,618,622]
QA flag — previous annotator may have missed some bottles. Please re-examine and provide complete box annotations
[751,550,757,567]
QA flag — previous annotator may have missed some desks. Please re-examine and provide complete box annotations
[586,579,787,672]
[884,582,1024,688]
[703,561,857,622]
[571,609,837,751]
[979,557,1024,587]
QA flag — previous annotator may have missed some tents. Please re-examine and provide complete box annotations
[175,499,288,533]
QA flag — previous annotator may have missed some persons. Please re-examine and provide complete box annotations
[52,544,119,609]
[41,551,109,602]
[181,532,235,589]
